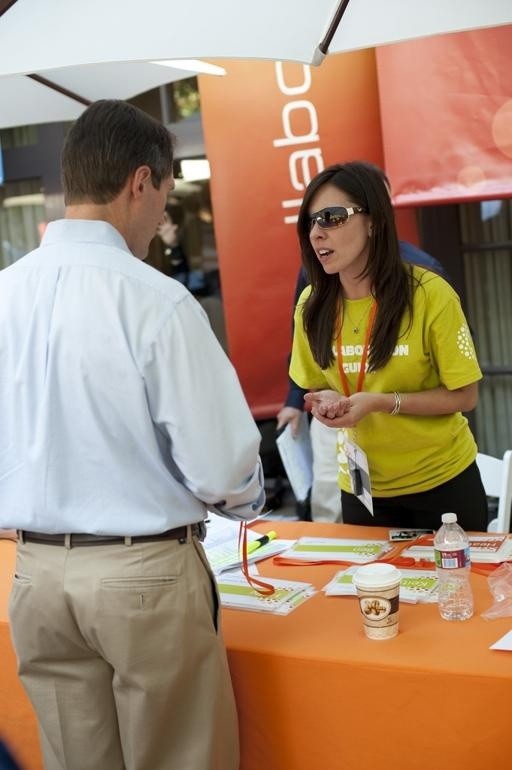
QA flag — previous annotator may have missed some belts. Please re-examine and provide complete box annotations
[17,525,201,544]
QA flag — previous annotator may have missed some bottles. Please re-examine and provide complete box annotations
[353,563,401,641]
[433,512,474,622]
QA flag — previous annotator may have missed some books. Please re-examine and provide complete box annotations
[199,511,299,575]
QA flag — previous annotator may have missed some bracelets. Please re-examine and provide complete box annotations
[390,390,401,418]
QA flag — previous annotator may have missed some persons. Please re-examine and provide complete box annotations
[0,96,271,767]
[273,236,446,519]
[286,167,496,534]
[157,201,190,295]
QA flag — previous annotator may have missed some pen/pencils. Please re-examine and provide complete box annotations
[246,530,276,553]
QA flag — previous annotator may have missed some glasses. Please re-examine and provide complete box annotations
[308,206,366,229]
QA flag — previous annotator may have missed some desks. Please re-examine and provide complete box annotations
[0,522,512,770]
